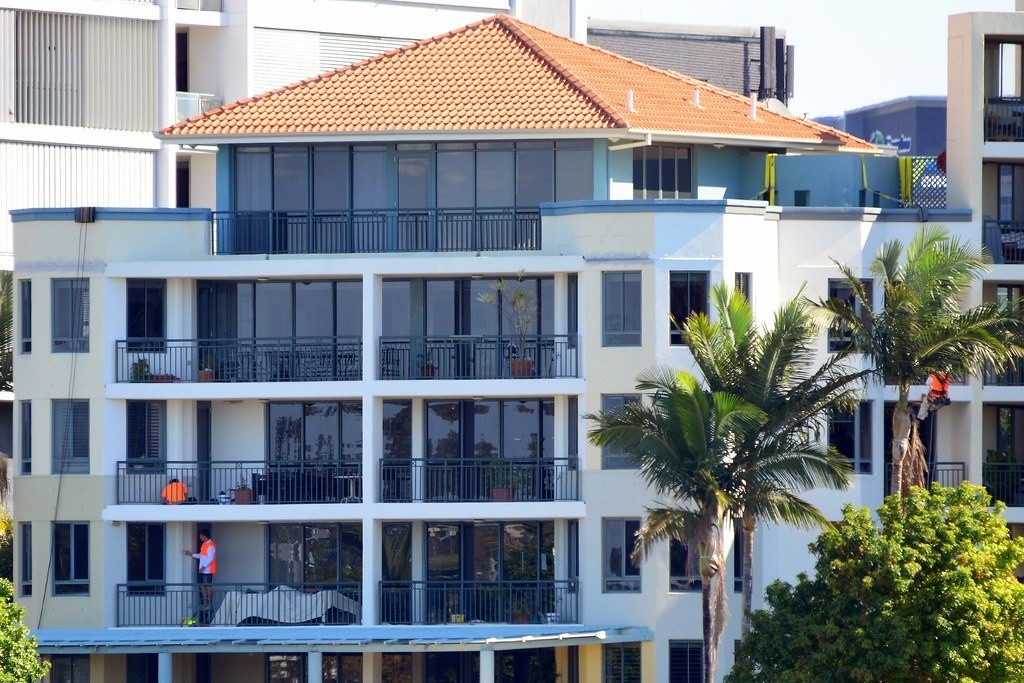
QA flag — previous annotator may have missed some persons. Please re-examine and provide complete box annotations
[916,364,952,424]
[162,479,188,505]
[183,527,218,612]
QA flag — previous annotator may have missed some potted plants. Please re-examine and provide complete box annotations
[199,353,214,383]
[126,358,180,383]
[476,270,539,381]
[233,473,252,505]
[501,551,543,624]
[488,457,521,501]
[412,349,434,378]
